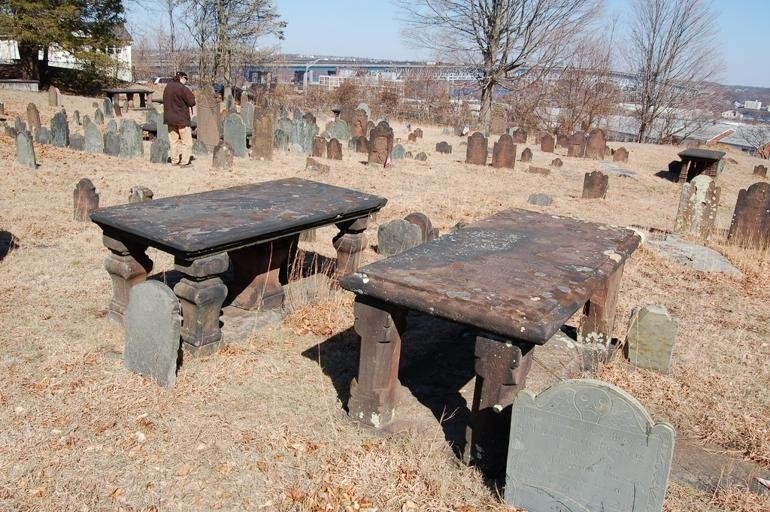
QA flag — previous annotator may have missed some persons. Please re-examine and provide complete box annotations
[163,71,196,169]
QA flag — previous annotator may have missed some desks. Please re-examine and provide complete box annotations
[338,206,646,467]
[87,177,388,346]
[677,148,726,183]
[101,88,155,110]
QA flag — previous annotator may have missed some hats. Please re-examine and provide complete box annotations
[177,71,189,80]
